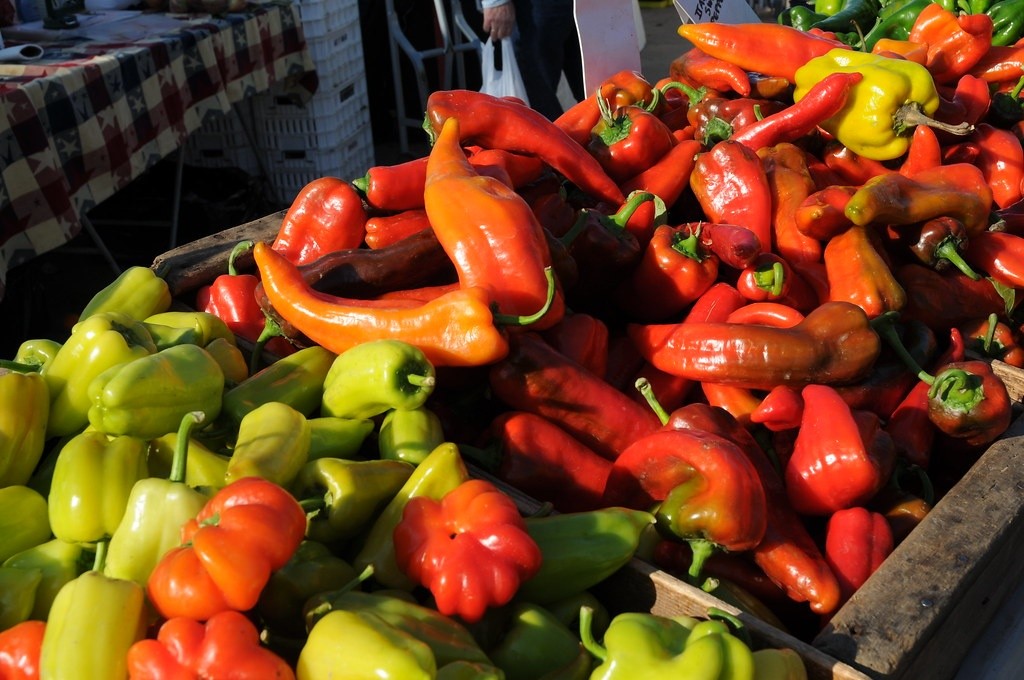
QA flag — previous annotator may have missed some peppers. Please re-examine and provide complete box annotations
[0,0,1024,680]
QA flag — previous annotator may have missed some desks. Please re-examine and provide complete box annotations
[0,0,299,276]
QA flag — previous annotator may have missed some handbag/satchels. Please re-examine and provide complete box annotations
[477,37,531,108]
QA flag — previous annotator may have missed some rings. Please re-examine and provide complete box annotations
[490,28,498,34]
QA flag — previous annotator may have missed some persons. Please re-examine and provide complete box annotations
[482,0,585,123]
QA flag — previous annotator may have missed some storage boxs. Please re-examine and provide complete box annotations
[142,211,1024,680]
[184,0,378,206]
